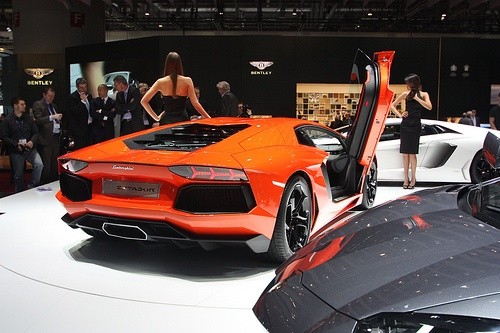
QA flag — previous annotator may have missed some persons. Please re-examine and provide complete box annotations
[90,84,117,144]
[212,82,253,118]
[329,112,354,129]
[0,97,44,192]
[114,77,162,137]
[140,52,212,126]
[33,87,63,184]
[390,74,432,189]
[459,110,477,126]
[67,78,93,148]
[186,88,201,118]
[489,104,500,130]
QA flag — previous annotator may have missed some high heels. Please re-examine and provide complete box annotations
[403,182,409,189]
[407,183,416,189]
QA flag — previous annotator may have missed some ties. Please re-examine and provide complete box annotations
[49,103,59,124]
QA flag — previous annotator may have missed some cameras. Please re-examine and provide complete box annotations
[19,143,31,153]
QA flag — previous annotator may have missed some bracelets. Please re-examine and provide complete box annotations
[250,114,252,116]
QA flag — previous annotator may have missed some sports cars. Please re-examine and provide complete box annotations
[252,176,500,333]
[56,48,395,266]
[310,118,500,184]
